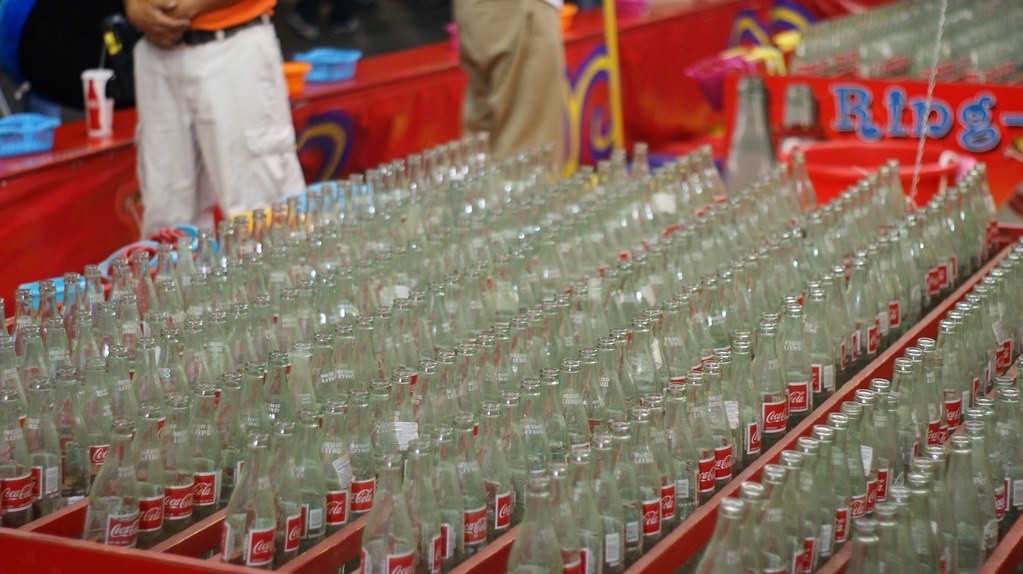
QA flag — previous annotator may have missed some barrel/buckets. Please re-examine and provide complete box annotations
[786,139,961,205]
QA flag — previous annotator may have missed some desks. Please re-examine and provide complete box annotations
[0,0,891,324]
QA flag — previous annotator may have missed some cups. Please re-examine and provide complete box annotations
[82,68,114,136]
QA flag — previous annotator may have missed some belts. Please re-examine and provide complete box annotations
[182,15,273,45]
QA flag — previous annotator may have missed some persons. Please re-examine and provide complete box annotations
[289,0,360,41]
[450,0,567,180]
[120,0,309,245]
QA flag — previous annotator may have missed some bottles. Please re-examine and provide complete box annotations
[1,132,1022,574]
[789,0,1023,89]
[726,78,771,185]
[778,84,820,158]
